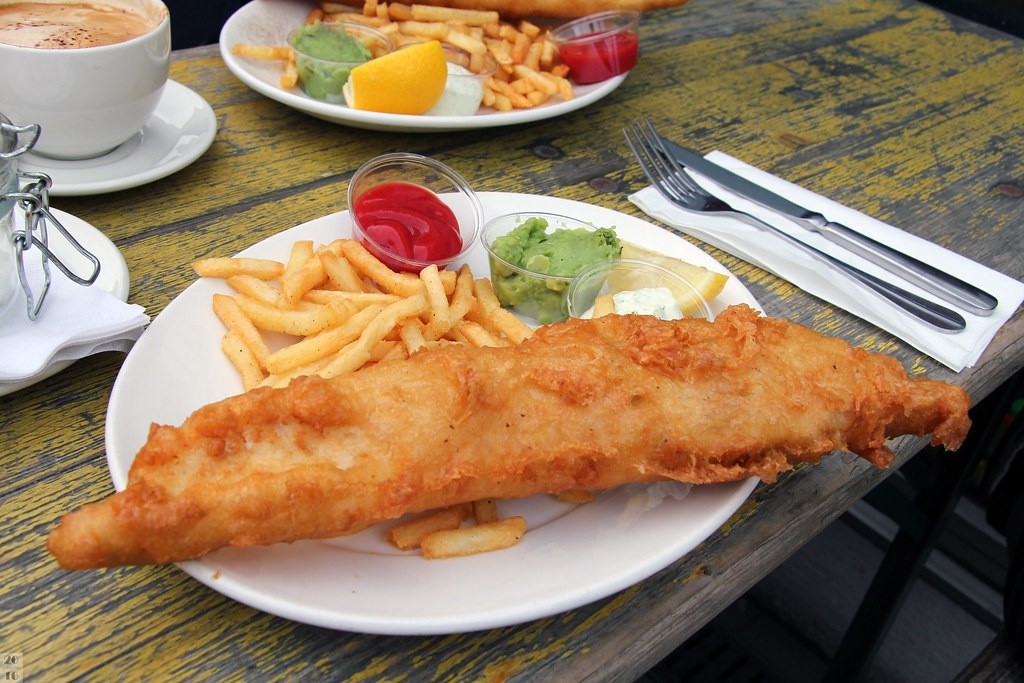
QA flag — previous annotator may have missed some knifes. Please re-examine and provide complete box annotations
[645,134,999,318]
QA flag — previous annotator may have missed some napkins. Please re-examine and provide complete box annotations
[0,272,151,383]
[627,148,1024,376]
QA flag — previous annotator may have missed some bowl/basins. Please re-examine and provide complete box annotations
[393,42,498,116]
[480,212,614,327]
[546,7,641,86]
[285,21,396,104]
[567,259,715,323]
[346,153,486,276]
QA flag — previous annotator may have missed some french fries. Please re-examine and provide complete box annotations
[190,235,615,560]
[232,0,574,112]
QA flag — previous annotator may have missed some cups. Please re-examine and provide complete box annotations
[0,0,171,160]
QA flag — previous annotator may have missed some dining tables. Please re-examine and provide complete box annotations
[0,0,1024,683]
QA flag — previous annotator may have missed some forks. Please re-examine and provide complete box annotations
[622,117,968,336]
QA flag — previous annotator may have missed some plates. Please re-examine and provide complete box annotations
[220,0,631,135]
[0,205,130,397]
[16,78,218,196]
[105,193,768,636]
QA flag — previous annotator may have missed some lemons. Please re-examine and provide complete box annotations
[343,39,448,115]
[606,239,730,318]
